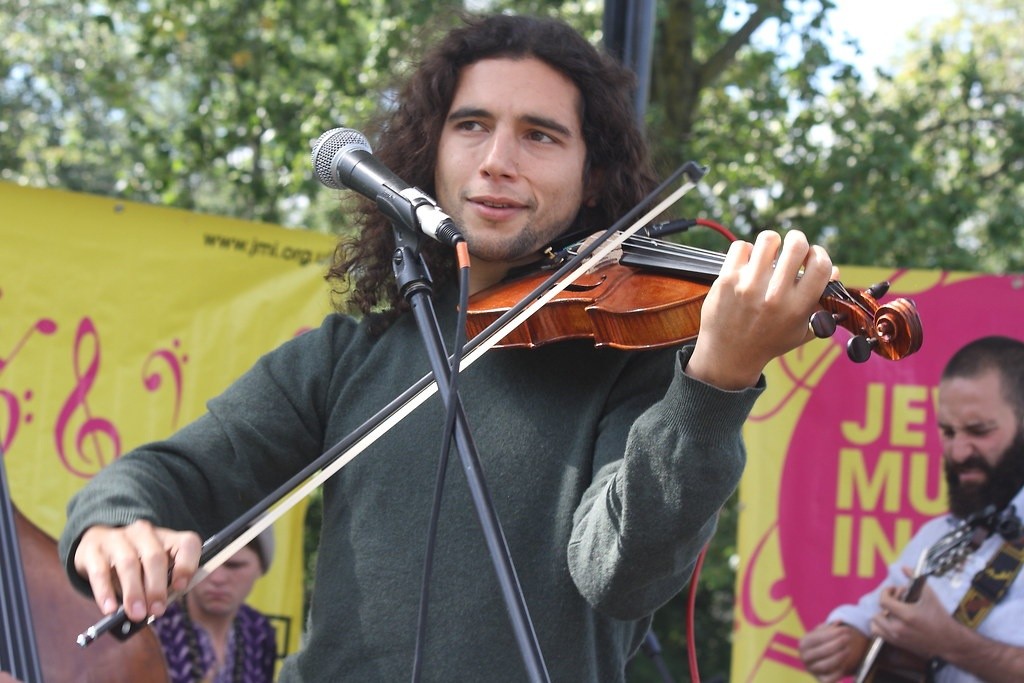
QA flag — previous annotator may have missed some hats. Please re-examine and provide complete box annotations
[247,510,275,576]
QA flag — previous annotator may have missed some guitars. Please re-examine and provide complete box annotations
[858,500,1007,683]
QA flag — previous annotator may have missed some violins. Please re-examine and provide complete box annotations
[457,231,924,366]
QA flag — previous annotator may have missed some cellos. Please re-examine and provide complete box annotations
[0,435,172,683]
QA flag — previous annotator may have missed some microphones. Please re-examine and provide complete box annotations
[312,127,463,247]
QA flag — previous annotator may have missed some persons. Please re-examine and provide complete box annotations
[123,510,277,683]
[58,13,839,683]
[798,335,1024,683]
